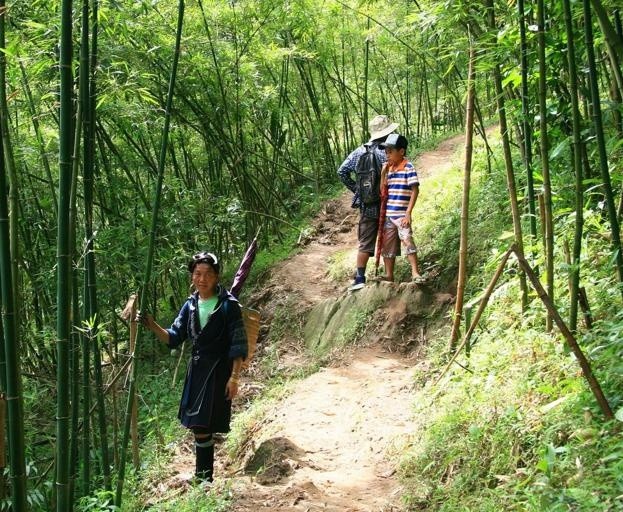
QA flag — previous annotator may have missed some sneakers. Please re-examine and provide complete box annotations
[348,276,365,290]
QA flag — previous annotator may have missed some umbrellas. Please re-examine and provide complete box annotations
[374,173,388,278]
[227,224,261,299]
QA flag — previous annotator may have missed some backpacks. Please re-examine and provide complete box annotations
[355,141,382,203]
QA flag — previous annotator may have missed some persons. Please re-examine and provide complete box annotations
[372,133,432,286]
[132,249,249,486]
[334,115,399,293]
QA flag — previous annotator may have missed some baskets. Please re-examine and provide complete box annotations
[242,307,261,369]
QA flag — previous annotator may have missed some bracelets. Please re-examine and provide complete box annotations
[228,367,240,384]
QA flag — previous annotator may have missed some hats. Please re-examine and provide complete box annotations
[368,115,400,142]
[378,134,408,150]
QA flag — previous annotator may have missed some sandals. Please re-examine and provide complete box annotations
[374,275,394,281]
[411,276,430,284]
